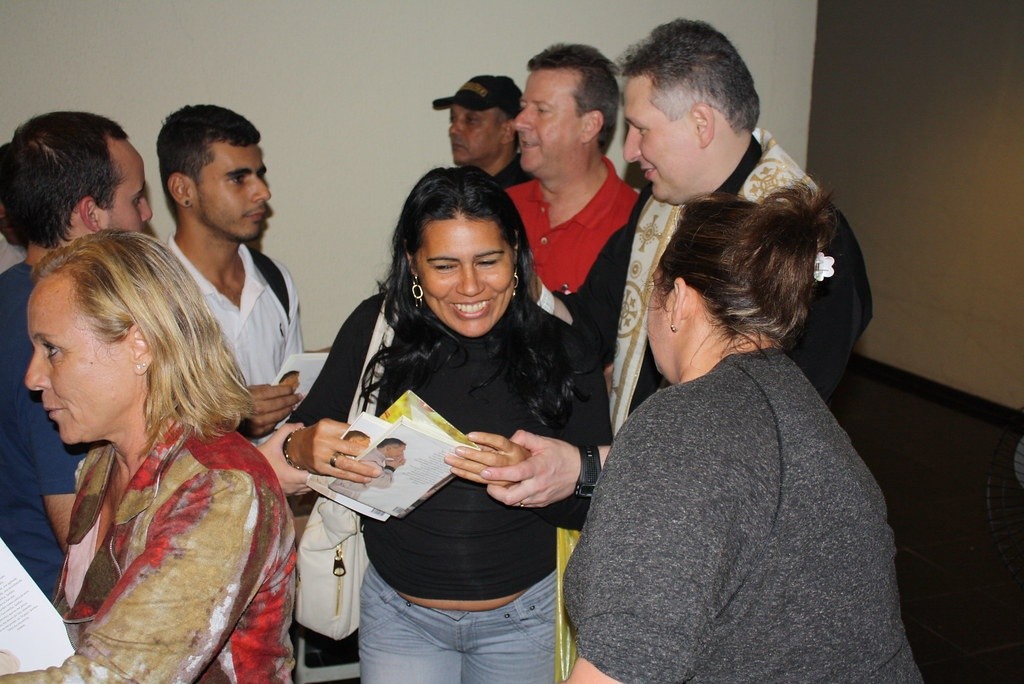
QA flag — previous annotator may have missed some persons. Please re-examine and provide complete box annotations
[331,438,406,501]
[156,104,304,447]
[307,431,370,500]
[431,74,536,189]
[565,182,924,683]
[0,112,153,603]
[0,228,296,683]
[281,166,614,683]
[503,43,640,294]
[480,19,875,522]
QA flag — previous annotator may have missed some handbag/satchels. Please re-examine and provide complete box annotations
[295,300,402,640]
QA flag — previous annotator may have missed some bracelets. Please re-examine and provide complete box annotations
[282,427,305,469]
[385,466,395,472]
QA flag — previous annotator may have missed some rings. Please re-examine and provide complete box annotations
[520,501,523,506]
[330,452,340,467]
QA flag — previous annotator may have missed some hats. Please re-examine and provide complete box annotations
[433,75,522,118]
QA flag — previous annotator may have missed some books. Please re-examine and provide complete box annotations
[303,390,481,522]
[0,537,75,675]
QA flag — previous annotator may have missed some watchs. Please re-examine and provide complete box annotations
[574,446,602,498]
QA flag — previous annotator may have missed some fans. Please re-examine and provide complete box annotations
[983,407,1024,588]
